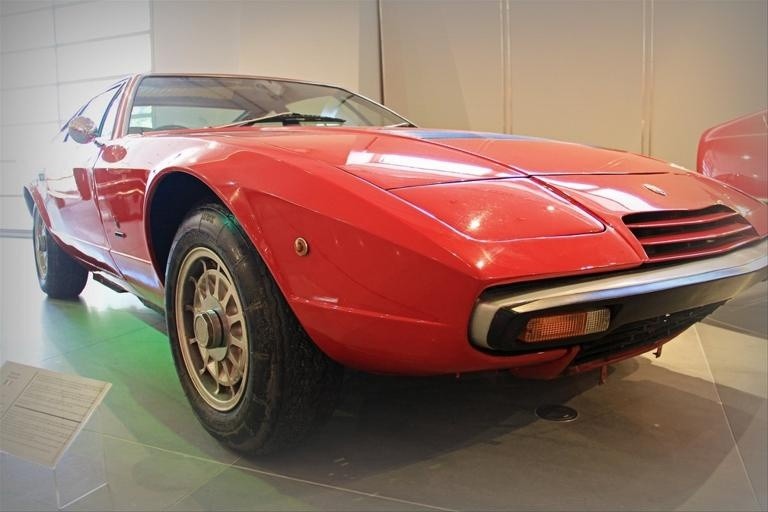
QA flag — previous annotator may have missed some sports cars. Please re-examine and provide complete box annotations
[14,73,766,452]
